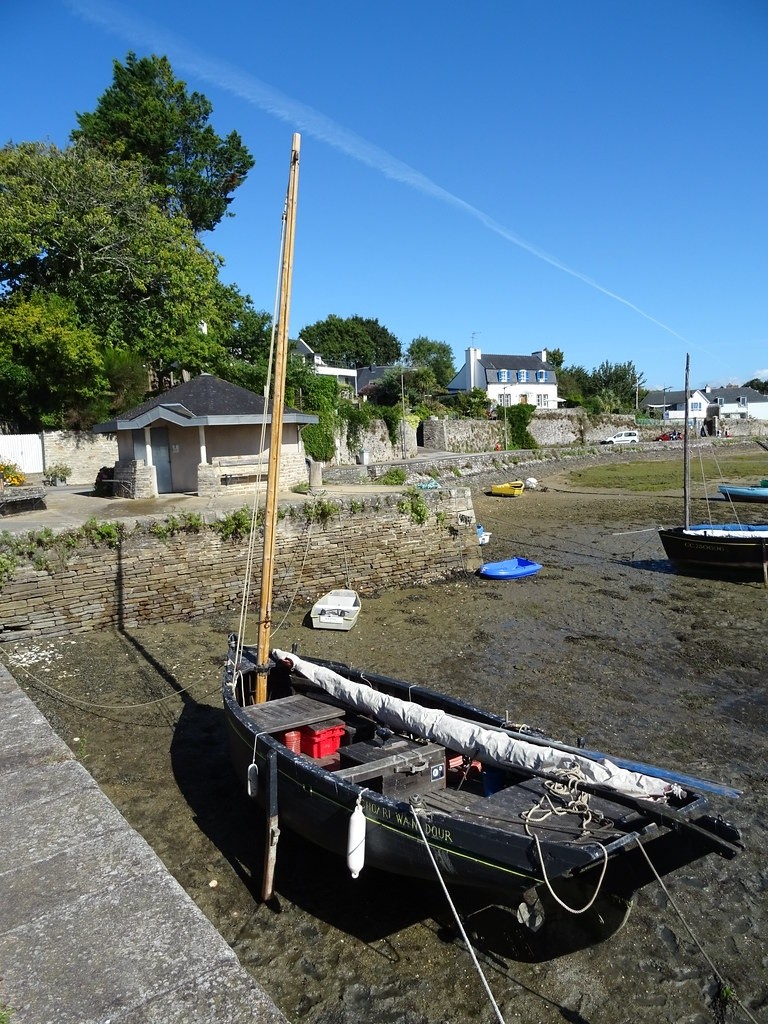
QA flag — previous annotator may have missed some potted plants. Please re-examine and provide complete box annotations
[42,462,72,487]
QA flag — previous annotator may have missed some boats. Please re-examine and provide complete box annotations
[719,484,768,501]
[309,588,361,632]
[493,481,524,497]
[659,355,768,581]
[479,555,543,579]
[230,132,750,949]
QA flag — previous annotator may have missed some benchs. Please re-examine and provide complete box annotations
[0,486,47,510]
[452,777,648,850]
[102,479,132,498]
[242,690,351,734]
[222,472,269,487]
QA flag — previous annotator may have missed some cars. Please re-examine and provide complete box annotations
[656,430,683,441]
[606,431,639,444]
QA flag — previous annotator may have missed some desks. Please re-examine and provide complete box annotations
[333,736,450,783]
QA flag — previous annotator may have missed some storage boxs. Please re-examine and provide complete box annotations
[301,718,345,758]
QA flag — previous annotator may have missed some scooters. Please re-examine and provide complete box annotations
[669,426,678,440]
